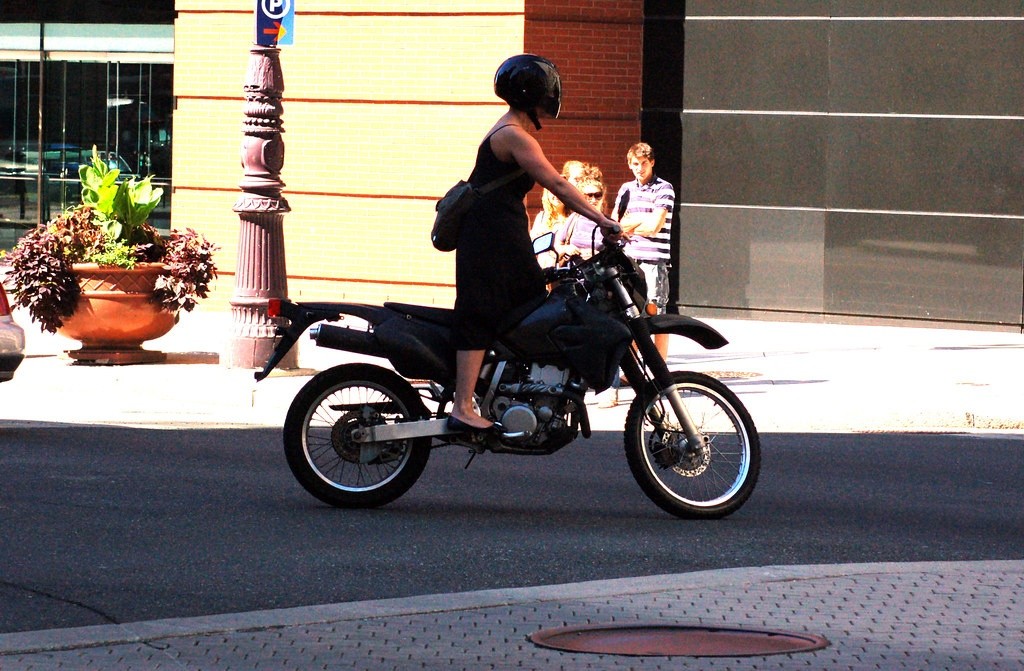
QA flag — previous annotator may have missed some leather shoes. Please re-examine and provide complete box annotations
[447,415,508,437]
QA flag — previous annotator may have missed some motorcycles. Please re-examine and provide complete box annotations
[251,188,765,521]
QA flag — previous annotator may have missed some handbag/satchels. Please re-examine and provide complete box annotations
[431,180,480,252]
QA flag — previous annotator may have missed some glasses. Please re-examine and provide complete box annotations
[584,192,602,198]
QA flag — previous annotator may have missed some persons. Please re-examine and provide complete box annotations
[531,160,591,296]
[611,143,675,388]
[554,181,620,408]
[446,55,632,433]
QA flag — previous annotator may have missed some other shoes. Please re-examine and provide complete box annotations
[584,385,619,408]
[612,377,631,389]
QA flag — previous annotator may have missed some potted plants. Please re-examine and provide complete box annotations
[0,144,223,364]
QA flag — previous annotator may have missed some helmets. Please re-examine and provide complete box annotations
[494,53,562,131]
